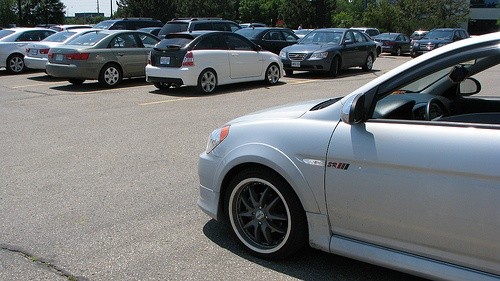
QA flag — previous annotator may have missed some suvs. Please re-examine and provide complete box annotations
[411,27,471,58]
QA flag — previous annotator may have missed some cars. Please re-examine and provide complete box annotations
[45,30,163,88]
[197,33,500,281]
[373,32,410,55]
[0,16,381,74]
[279,28,377,78]
[146,32,283,94]
